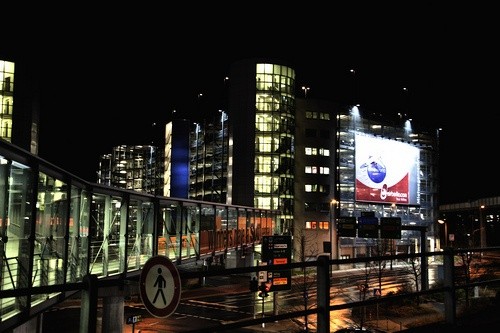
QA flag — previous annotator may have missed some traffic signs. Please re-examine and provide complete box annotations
[335,217,402,240]
[261,236,292,290]
